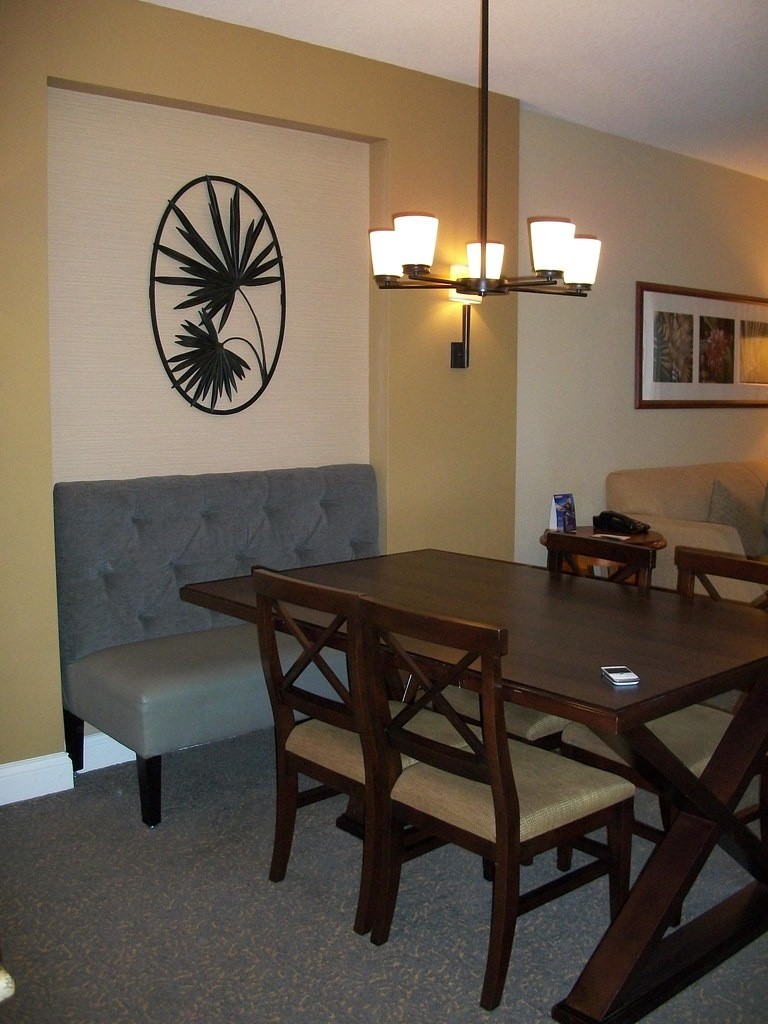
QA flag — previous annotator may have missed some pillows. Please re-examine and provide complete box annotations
[708,476,768,559]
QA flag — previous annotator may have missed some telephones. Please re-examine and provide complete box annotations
[593,510,650,535]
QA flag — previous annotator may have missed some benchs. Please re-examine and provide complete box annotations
[52,461,379,830]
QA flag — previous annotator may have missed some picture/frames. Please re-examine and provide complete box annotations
[632,279,768,409]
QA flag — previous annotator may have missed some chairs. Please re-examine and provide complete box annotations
[534,543,768,928]
[250,566,494,936]
[440,530,656,866]
[350,594,638,1012]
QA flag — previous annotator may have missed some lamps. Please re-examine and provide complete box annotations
[445,259,483,371]
[359,1,604,305]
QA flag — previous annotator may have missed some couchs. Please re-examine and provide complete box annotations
[603,459,768,605]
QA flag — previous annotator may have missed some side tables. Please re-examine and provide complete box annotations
[540,526,667,585]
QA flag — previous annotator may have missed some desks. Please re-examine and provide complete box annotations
[180,548,768,1024]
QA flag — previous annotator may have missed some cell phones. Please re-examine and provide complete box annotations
[600,666,640,685]
